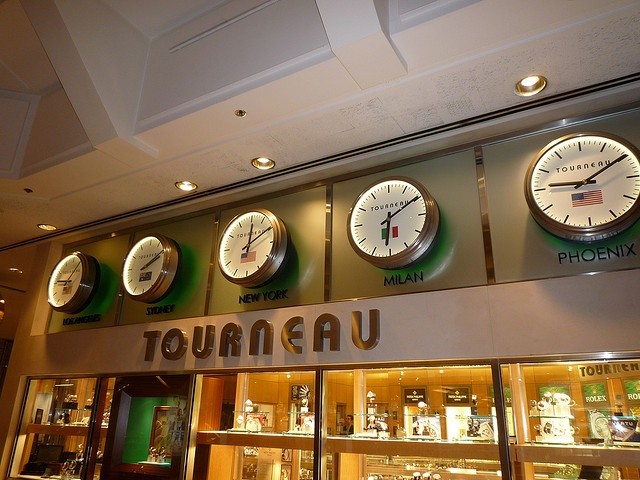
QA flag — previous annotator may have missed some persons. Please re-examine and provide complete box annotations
[340,414,354,435]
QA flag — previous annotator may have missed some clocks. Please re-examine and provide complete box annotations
[523,131,640,242]
[218,208,288,288]
[47,251,99,314]
[121,234,178,303]
[346,175,439,270]
